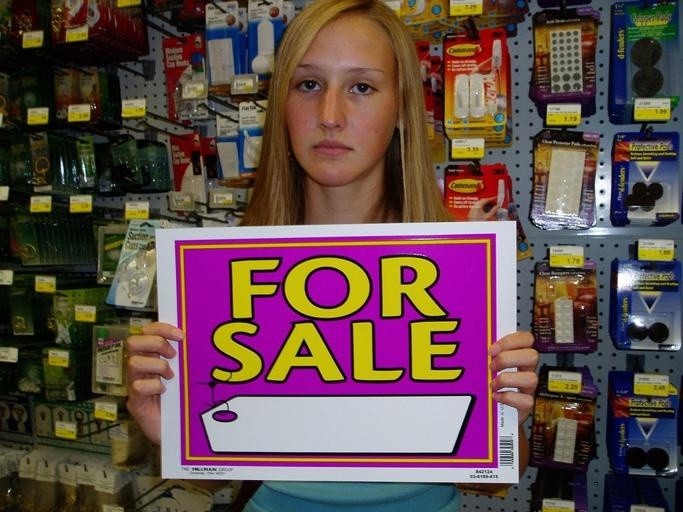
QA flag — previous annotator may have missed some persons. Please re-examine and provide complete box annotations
[124,2,540,512]
